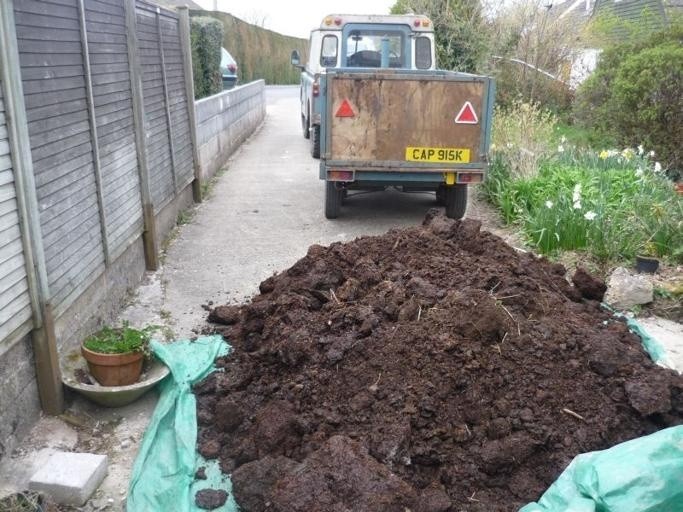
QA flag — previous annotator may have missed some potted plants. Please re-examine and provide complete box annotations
[635,242,661,276]
[80,318,150,387]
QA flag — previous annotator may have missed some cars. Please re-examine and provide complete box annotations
[219,47,237,89]
